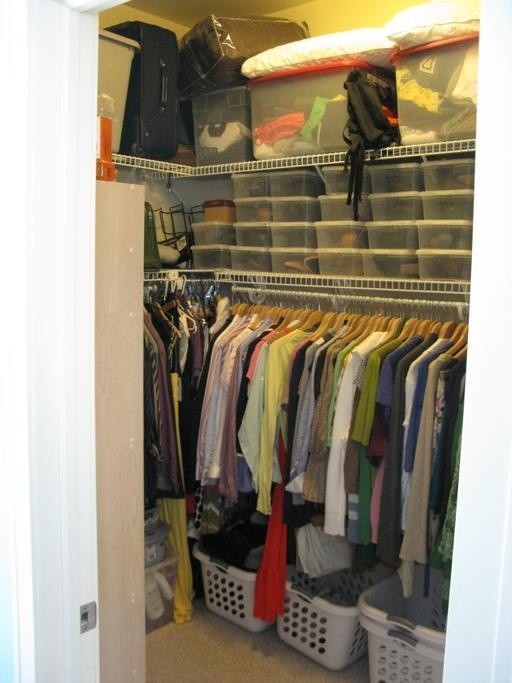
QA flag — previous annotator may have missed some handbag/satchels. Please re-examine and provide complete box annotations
[179,13,310,101]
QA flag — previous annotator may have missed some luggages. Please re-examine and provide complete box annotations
[104,21,180,160]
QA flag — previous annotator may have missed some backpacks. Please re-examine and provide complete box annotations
[342,69,401,220]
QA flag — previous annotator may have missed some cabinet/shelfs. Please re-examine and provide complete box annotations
[95,134,475,296]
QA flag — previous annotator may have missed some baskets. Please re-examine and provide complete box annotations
[357,562,445,683]
[276,560,396,671]
[192,541,277,633]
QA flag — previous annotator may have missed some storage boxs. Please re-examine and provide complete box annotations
[145,506,180,635]
